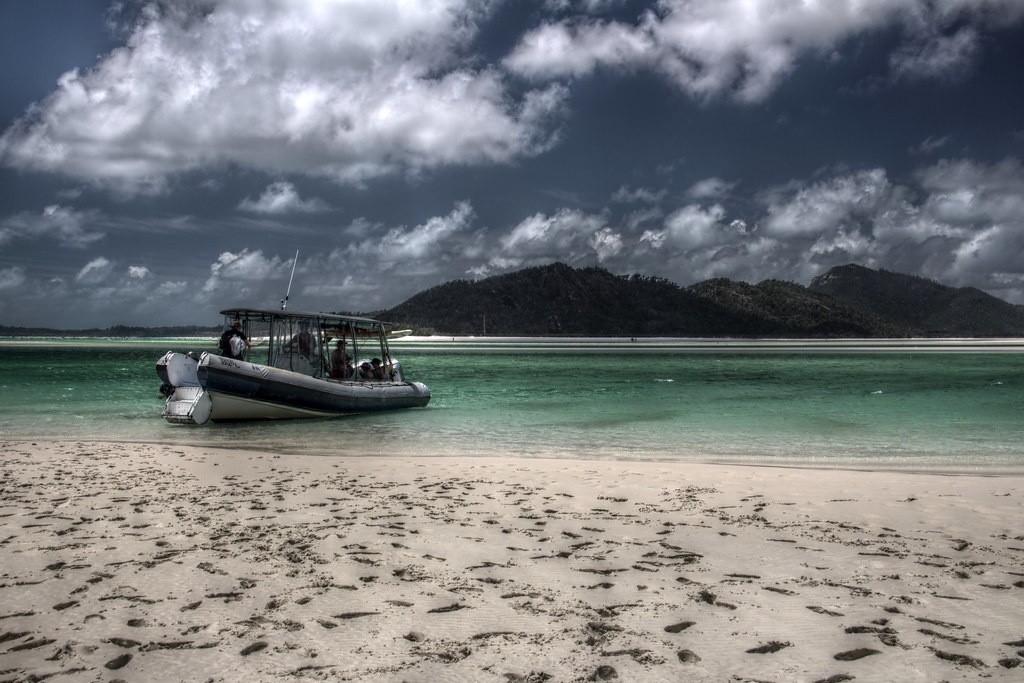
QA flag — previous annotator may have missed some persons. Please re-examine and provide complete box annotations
[371,357,384,381]
[282,320,333,375]
[218,321,260,362]
[359,362,375,378]
[332,338,353,379]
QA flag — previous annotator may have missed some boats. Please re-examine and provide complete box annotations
[319,327,413,338]
[155,249,432,427]
